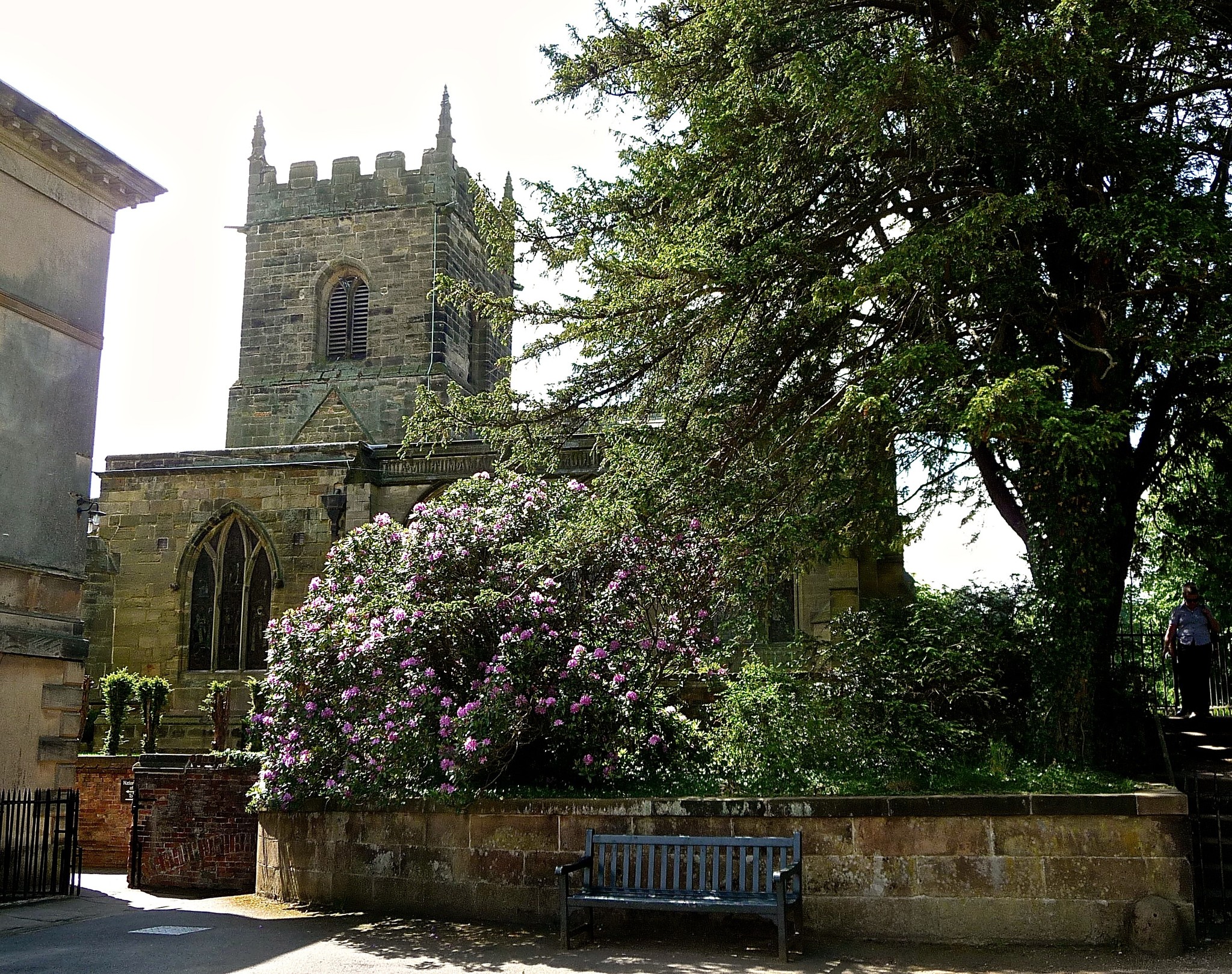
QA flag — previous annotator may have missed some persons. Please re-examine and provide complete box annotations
[1163,581,1220,717]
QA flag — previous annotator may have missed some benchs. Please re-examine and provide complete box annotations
[554,828,805,964]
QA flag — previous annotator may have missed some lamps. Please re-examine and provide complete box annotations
[76,499,107,533]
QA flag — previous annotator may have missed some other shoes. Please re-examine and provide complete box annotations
[1178,708,1188,716]
[1205,712,1212,718]
